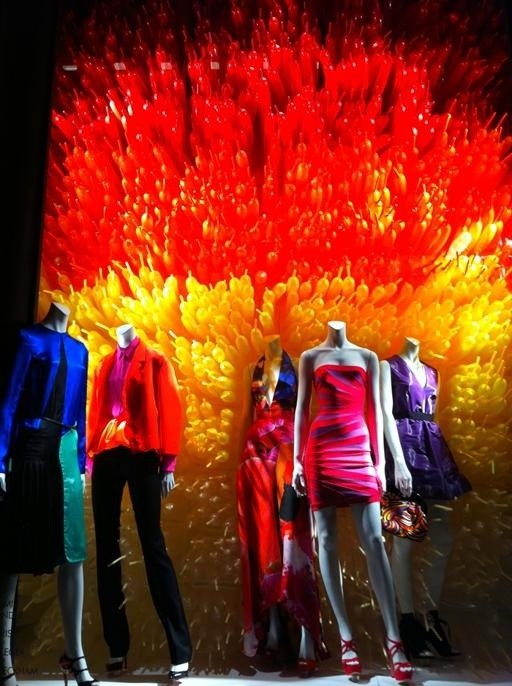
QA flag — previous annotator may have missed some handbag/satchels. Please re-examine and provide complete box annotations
[380,481,433,544]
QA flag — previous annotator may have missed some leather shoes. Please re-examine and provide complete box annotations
[167,661,190,680]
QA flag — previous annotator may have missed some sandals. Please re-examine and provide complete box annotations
[381,633,414,683]
[336,636,363,684]
[0,667,21,686]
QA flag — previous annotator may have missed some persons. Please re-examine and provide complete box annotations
[381,333,476,661]
[85,323,193,679]
[0,300,100,685]
[232,333,333,674]
[291,320,417,683]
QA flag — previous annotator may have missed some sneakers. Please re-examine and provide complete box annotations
[260,645,319,674]
[420,608,463,660]
[397,612,437,663]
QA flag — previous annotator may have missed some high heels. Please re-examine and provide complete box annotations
[105,654,129,672]
[59,647,101,686]
